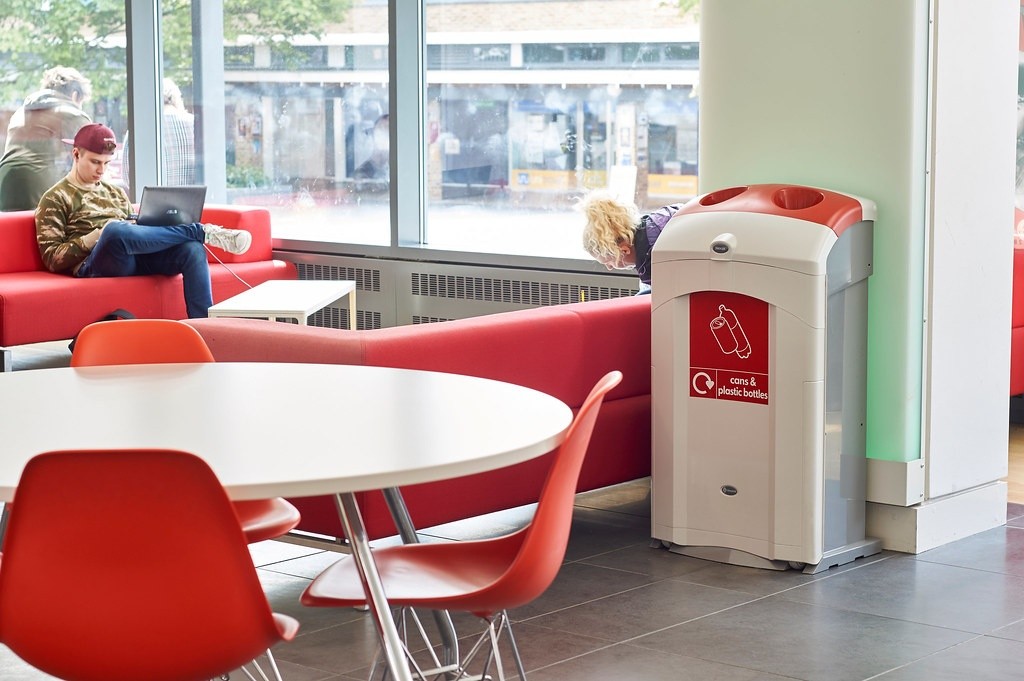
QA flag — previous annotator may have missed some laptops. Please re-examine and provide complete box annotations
[135,184,208,226]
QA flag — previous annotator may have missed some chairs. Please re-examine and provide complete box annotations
[71,319,302,547]
[298,368,624,681]
[0,451,301,681]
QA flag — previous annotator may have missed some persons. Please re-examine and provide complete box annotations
[582,197,687,295]
[0,65,95,212]
[122,77,195,197]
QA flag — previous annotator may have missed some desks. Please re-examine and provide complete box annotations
[0,362,574,680]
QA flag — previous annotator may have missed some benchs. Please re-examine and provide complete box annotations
[176,295,651,609]
[0,203,297,376]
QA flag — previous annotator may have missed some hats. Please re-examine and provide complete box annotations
[61,123,117,156]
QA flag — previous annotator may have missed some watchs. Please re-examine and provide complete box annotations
[35,122,253,319]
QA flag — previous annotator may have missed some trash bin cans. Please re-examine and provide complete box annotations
[651,180,884,573]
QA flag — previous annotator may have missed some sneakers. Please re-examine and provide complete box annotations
[200,222,251,255]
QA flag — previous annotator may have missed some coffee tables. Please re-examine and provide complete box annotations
[209,280,357,331]
